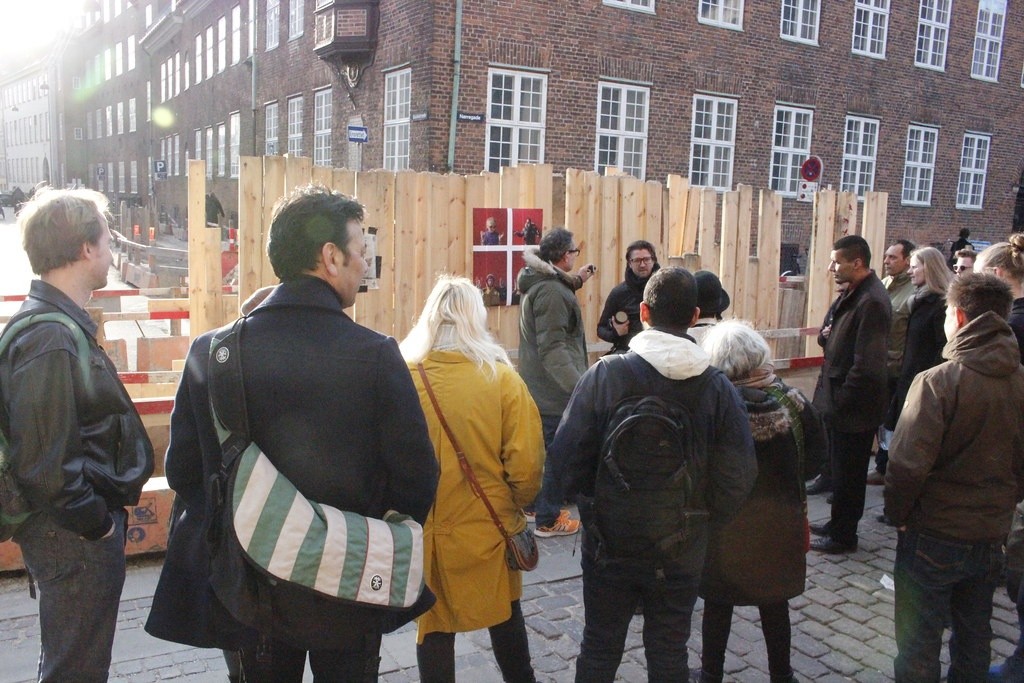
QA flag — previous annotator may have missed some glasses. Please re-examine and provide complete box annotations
[564,248,580,258]
[489,226,495,228]
[629,257,652,264]
[953,263,974,272]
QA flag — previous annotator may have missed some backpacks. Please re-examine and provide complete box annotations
[0,313,96,543]
[589,352,721,580]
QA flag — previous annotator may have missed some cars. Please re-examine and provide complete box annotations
[0,192,13,207]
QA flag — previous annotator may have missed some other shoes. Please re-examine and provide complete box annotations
[806,477,830,494]
[988,664,1008,683]
[826,491,834,503]
[866,472,886,485]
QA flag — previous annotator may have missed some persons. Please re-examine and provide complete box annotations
[544,265,758,683]
[144,182,441,683]
[698,319,830,683]
[475,273,506,306]
[480,216,504,245]
[0,189,154,683]
[516,227,596,537]
[514,217,540,245]
[206,192,225,227]
[685,271,730,343]
[808,229,1024,683]
[13,187,24,203]
[597,241,661,355]
[399,275,545,683]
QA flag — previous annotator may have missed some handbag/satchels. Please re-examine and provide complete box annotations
[505,532,538,571]
[204,444,424,654]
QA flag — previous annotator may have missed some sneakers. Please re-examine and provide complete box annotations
[524,506,570,525]
[534,509,581,539]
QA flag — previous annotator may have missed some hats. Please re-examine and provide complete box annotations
[690,271,730,313]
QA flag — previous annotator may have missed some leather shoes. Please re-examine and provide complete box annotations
[808,523,829,535]
[809,532,859,553]
